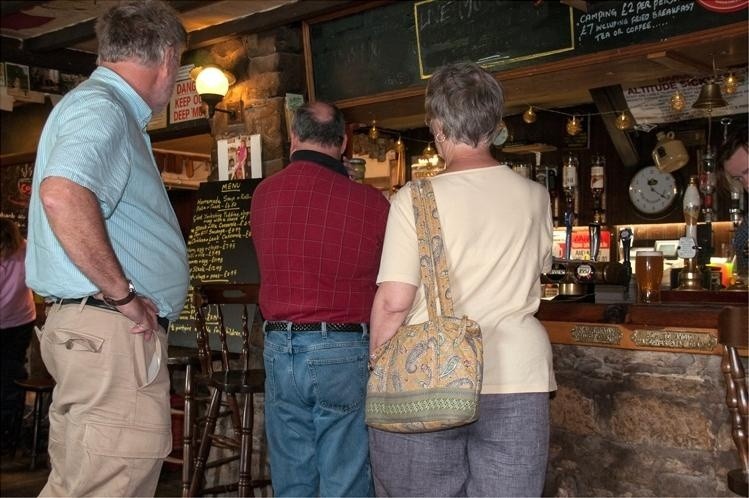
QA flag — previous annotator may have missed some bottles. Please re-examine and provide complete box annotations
[589,156,605,211]
[705,266,722,290]
[499,160,547,188]
[562,156,579,222]
[352,159,366,184]
[696,143,718,211]
[548,165,561,220]
[546,284,559,296]
[728,177,742,213]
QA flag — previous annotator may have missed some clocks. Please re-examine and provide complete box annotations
[624,160,683,222]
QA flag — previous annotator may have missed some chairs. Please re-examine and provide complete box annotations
[717,306,749,497]
[185,279,286,498]
[155,344,211,497]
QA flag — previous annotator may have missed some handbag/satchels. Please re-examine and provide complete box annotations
[364,314,483,434]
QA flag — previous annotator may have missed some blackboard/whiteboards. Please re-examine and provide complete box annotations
[0,151,37,239]
[298,0,749,110]
[166,178,264,354]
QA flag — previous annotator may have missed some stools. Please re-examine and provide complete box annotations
[12,375,56,472]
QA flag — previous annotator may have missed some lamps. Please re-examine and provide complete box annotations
[194,67,244,126]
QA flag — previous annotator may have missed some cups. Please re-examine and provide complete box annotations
[722,243,738,276]
[636,251,664,304]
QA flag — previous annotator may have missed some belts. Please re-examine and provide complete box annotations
[264,321,370,333]
[55,295,170,335]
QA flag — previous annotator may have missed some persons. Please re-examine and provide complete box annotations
[24,1,190,498]
[368,59,558,498]
[228,159,235,180]
[231,140,247,180]
[715,127,748,267]
[0,218,37,473]
[250,102,391,497]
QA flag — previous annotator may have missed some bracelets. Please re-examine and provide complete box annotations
[104,279,136,305]
